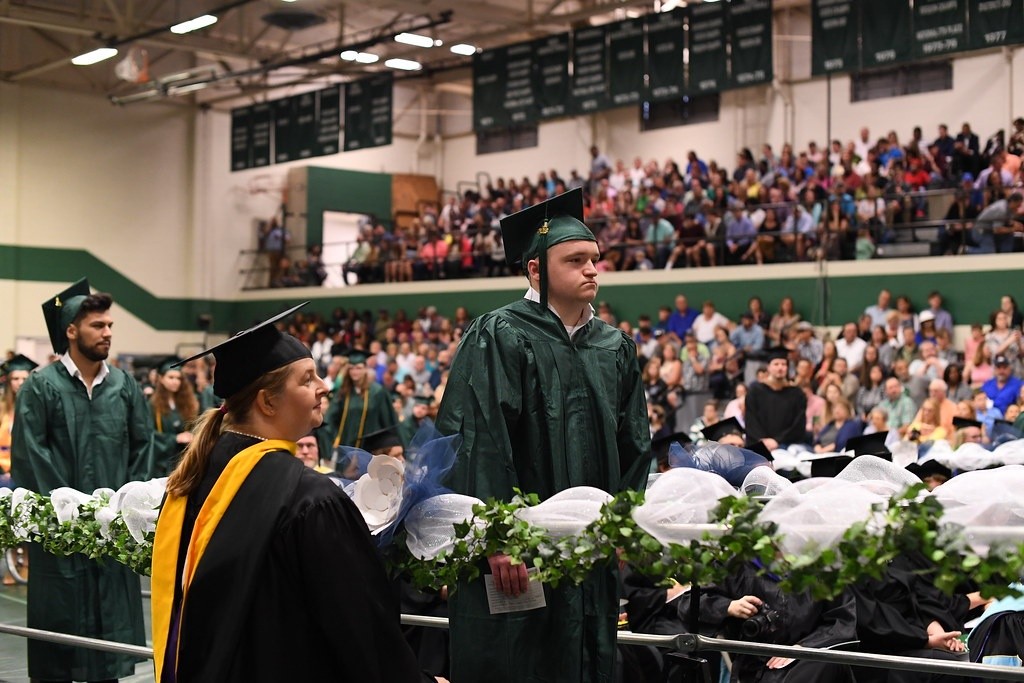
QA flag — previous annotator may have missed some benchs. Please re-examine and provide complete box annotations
[871,193,961,258]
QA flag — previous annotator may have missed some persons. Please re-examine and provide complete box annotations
[276,308,470,477]
[434,186,652,683]
[151,301,449,683]
[259,221,327,286]
[136,354,225,478]
[0,277,152,683]
[595,288,1024,683]
[342,118,1024,284]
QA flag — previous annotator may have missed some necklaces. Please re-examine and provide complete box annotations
[226,430,268,441]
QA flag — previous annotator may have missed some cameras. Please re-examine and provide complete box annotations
[742,605,780,636]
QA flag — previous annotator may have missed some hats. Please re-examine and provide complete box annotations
[700,415,746,442]
[166,300,316,399]
[742,440,774,461]
[775,466,810,482]
[343,348,375,364]
[305,421,329,467]
[952,416,983,429]
[993,354,1011,368]
[761,343,791,362]
[42,276,92,356]
[0,353,39,393]
[651,431,692,465]
[800,455,853,478]
[904,462,921,479]
[844,430,893,463]
[917,460,956,479]
[155,355,185,375]
[499,186,599,313]
[919,310,938,325]
[413,395,435,405]
